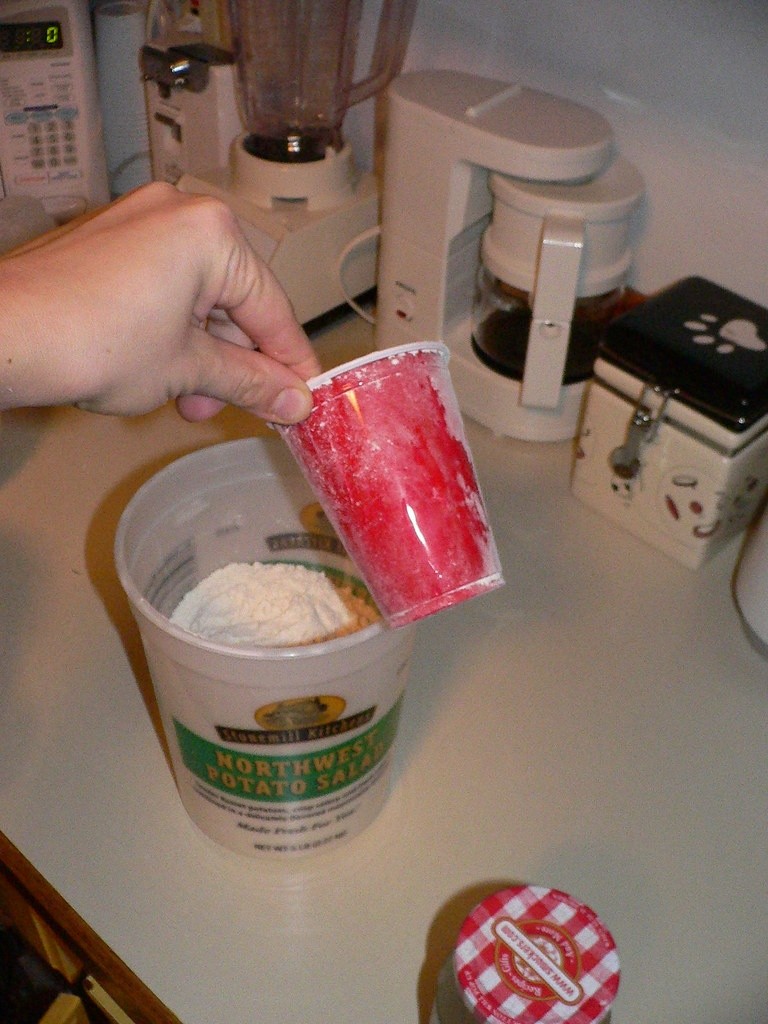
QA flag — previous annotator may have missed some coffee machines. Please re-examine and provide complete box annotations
[374,69,645,443]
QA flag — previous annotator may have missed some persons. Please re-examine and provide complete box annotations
[2,178,324,426]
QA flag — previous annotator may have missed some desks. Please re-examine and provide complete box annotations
[0,288,768,1024]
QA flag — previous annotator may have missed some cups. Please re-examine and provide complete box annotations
[265,337,507,632]
[113,429,416,859]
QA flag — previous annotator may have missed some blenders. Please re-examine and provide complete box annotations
[140,0,419,331]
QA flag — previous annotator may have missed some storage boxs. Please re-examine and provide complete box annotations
[572,275,768,568]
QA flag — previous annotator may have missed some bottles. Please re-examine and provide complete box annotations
[430,884,621,1023]
